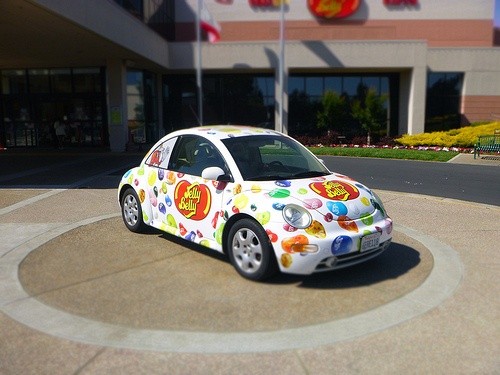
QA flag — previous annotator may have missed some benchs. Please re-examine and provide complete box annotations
[474,134,500,159]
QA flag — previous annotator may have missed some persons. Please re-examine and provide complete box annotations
[53,117,67,151]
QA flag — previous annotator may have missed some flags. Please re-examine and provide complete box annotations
[199,0,222,45]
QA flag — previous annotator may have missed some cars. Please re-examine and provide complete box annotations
[116,124,394,282]
